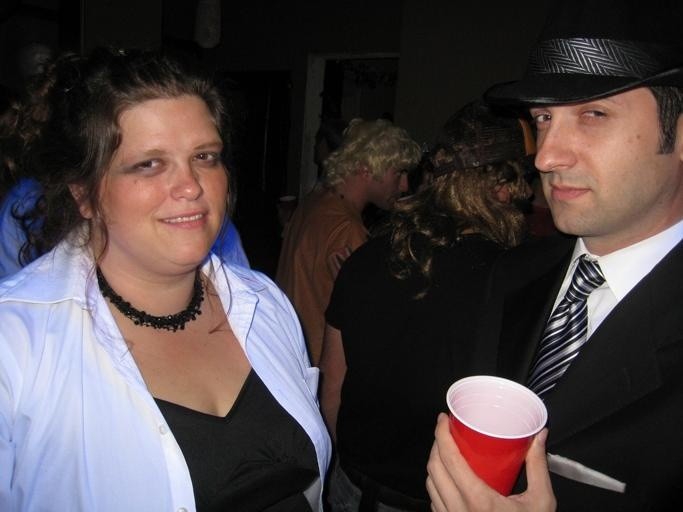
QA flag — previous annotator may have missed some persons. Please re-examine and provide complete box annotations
[267,117,349,262]
[0,174,255,283]
[425,0,683,509]
[0,31,333,510]
[312,93,558,510]
[272,113,423,363]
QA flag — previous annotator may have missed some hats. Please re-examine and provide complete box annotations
[487,2,681,105]
[429,98,536,178]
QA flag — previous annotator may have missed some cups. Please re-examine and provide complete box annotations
[446,375,548,498]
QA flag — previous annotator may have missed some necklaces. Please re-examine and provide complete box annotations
[94,264,206,333]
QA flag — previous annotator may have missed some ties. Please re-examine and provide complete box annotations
[520,252,605,404]
